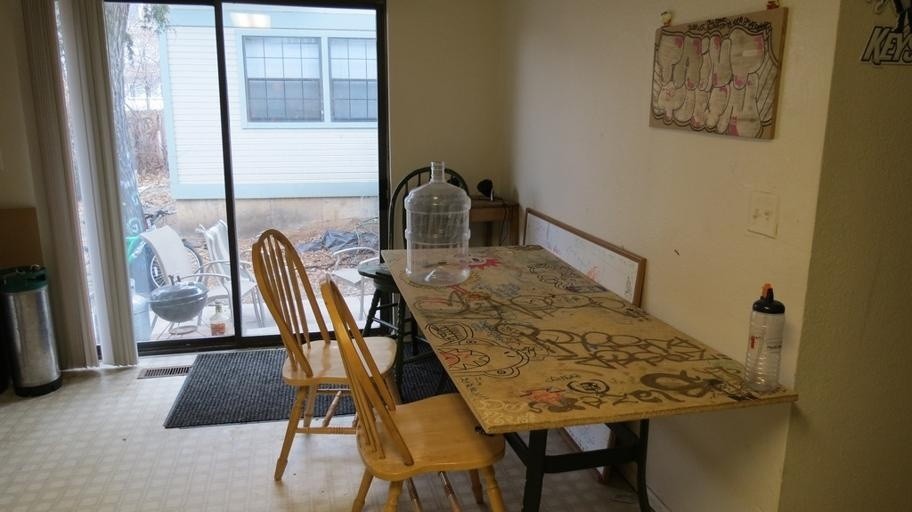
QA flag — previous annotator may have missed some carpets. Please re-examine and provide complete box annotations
[163,343,458,429]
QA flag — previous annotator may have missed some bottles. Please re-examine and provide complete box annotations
[741,281,787,392]
[208,302,229,336]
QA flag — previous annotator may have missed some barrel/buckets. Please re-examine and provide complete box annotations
[404,161,472,287]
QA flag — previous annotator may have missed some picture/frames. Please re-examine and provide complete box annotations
[523,207,646,481]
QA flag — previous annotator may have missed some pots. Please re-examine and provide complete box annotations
[147,275,210,323]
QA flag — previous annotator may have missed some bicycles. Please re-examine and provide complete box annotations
[82,210,203,299]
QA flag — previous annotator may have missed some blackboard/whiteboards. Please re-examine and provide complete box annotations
[520,204,648,482]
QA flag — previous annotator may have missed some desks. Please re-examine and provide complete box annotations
[381,245,798,512]
[469,196,519,245]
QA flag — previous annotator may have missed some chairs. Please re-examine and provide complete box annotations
[357,165,470,389]
[252,228,398,481]
[332,247,376,319]
[140,220,265,340]
[318,273,509,511]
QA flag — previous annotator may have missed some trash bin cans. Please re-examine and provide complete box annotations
[1,264,61,397]
